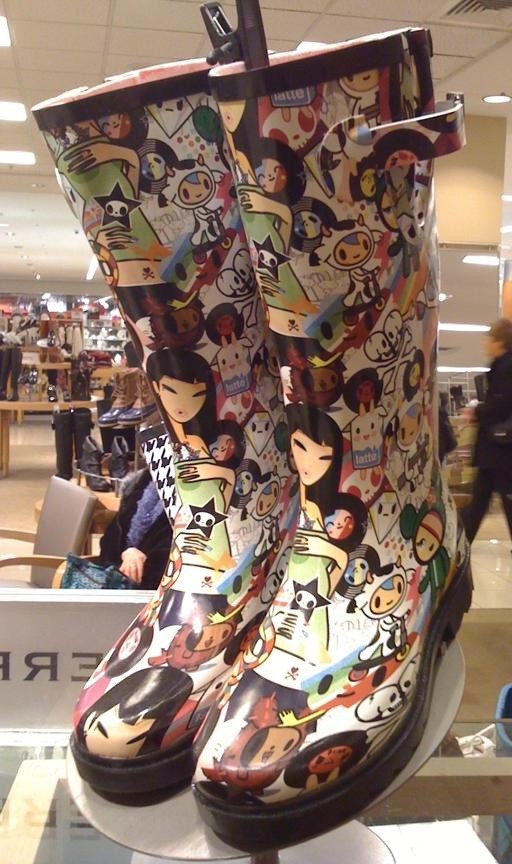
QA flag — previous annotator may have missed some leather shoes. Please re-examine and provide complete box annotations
[47,331,55,346]
[46,386,58,402]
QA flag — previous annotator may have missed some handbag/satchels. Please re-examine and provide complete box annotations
[61,550,136,589]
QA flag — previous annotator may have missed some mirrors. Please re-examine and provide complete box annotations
[434,240,504,517]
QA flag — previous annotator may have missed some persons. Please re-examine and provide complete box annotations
[436,394,457,467]
[461,316,512,547]
[96,463,174,592]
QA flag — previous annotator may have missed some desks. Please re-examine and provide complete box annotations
[0,400,97,478]
[34,487,121,536]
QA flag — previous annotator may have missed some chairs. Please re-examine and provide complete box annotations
[0,473,100,593]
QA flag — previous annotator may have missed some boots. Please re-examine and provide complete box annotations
[28,55,297,808]
[192,28,474,844]
[53,367,156,491]
[0,346,38,400]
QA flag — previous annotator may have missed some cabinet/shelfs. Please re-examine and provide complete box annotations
[1,316,133,394]
